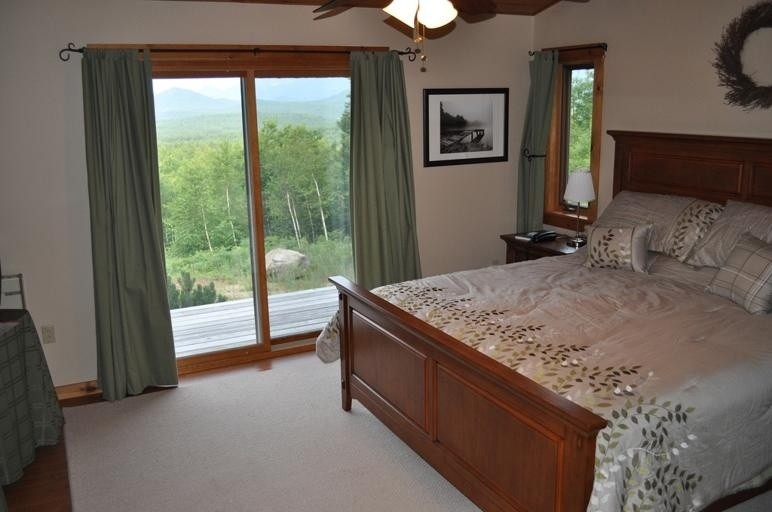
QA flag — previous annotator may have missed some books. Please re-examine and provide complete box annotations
[514,231,541,242]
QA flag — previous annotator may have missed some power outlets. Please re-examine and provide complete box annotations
[40,324,56,345]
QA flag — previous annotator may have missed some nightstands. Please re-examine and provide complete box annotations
[499,229,582,264]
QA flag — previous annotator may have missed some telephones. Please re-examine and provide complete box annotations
[531,230,557,243]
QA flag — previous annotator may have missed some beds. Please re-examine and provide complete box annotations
[326,129,772,512]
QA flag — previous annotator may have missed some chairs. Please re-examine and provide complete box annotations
[0,272,24,310]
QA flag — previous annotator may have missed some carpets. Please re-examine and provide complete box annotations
[60,356,481,512]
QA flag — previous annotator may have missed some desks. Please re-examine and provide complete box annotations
[0,309,65,487]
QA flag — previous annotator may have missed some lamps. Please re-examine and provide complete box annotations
[562,172,597,249]
[381,1,463,46]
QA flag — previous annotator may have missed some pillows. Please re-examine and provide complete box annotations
[683,196,772,268]
[703,231,772,316]
[581,223,653,273]
[592,189,723,264]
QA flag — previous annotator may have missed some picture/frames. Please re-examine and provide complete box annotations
[422,87,510,168]
